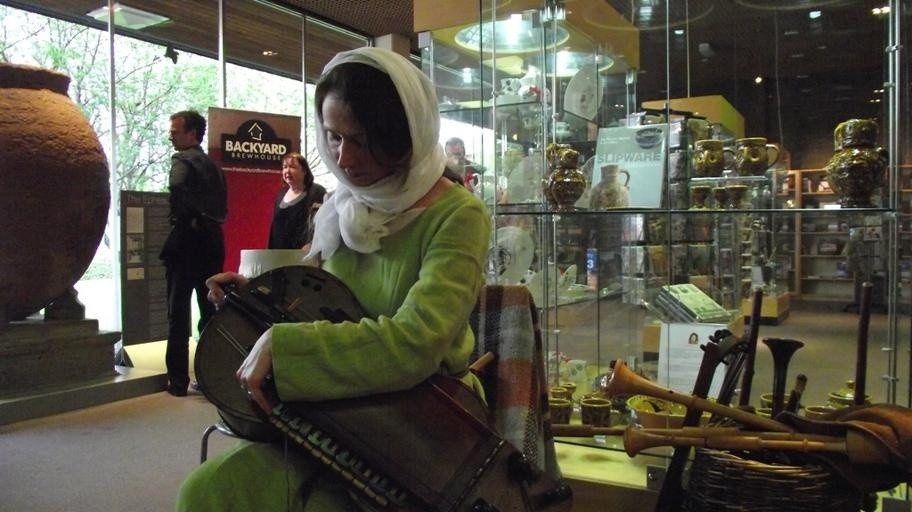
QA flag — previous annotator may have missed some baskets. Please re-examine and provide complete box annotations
[686,446,853,512]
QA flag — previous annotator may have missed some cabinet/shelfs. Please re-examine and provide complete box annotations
[424,7,632,408]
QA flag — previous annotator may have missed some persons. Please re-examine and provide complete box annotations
[267,151,329,250]
[176,45,490,512]
[156,108,227,396]
[444,137,488,173]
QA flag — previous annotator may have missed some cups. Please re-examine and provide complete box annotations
[561,382,577,399]
[580,397,622,428]
[688,184,749,210]
[547,397,572,426]
[550,387,568,401]
[733,136,780,176]
[691,139,736,177]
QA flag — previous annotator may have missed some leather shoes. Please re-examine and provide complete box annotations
[191,381,202,390]
[167,384,187,396]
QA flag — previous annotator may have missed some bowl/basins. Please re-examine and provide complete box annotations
[626,393,718,429]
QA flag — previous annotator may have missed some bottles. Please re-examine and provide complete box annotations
[589,164,632,208]
[549,149,587,208]
[824,118,890,209]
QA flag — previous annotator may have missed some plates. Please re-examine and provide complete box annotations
[563,66,605,120]
